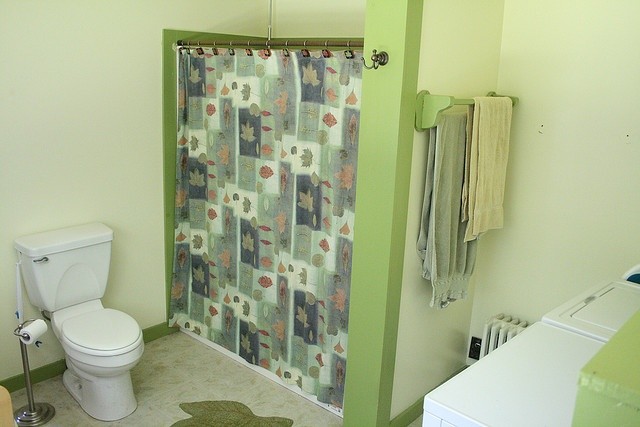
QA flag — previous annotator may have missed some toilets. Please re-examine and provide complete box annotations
[13,221,144,421]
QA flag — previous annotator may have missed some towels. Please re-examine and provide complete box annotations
[463,94,513,244]
[416,103,478,308]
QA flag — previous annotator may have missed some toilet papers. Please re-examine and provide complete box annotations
[19,318,48,345]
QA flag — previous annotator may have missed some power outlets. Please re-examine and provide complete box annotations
[468,336,482,360]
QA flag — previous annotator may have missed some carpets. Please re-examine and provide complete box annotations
[171,399,294,426]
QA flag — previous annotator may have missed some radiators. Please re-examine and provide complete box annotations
[481,312,531,358]
